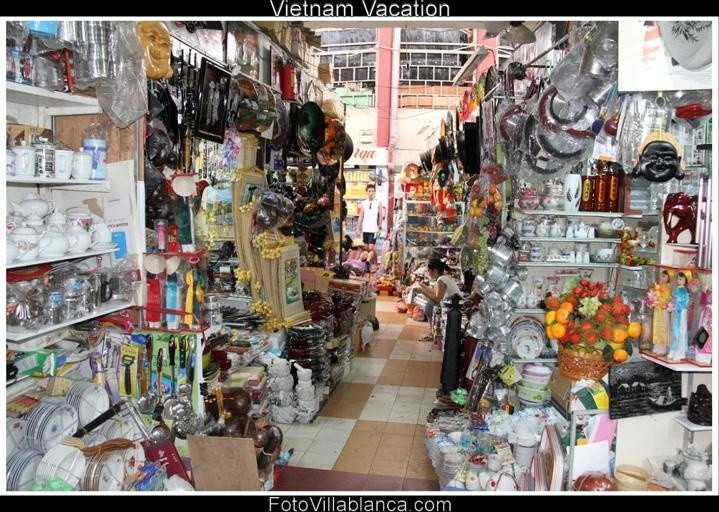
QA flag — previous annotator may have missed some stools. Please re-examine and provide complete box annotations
[360,294,378,331]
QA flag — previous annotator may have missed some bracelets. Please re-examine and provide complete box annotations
[379,227,383,230]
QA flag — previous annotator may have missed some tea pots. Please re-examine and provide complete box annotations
[6,194,117,263]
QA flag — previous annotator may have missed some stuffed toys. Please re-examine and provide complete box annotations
[648,266,676,357]
[666,268,696,363]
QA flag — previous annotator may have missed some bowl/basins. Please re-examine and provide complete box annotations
[295,369,318,413]
[517,361,552,407]
[512,175,627,267]
[425,415,466,490]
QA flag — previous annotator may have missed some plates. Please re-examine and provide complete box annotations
[507,316,547,360]
[7,383,147,490]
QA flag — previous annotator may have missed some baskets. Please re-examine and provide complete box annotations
[557,345,612,382]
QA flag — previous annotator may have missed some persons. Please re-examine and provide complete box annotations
[414,257,462,342]
[630,131,685,183]
[356,184,386,266]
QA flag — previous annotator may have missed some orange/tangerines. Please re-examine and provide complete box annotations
[547,302,573,339]
[613,322,641,362]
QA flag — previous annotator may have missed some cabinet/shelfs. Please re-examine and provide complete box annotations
[402,198,712,432]
[344,165,389,243]
[7,57,269,492]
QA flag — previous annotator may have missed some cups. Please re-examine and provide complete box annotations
[516,267,619,312]
[7,267,113,333]
[7,137,108,183]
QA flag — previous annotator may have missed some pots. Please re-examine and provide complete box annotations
[293,100,327,157]
[499,36,619,171]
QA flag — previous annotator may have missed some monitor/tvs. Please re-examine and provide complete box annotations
[192,57,232,145]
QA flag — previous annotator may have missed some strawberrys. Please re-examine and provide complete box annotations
[549,298,560,308]
[558,279,630,343]
